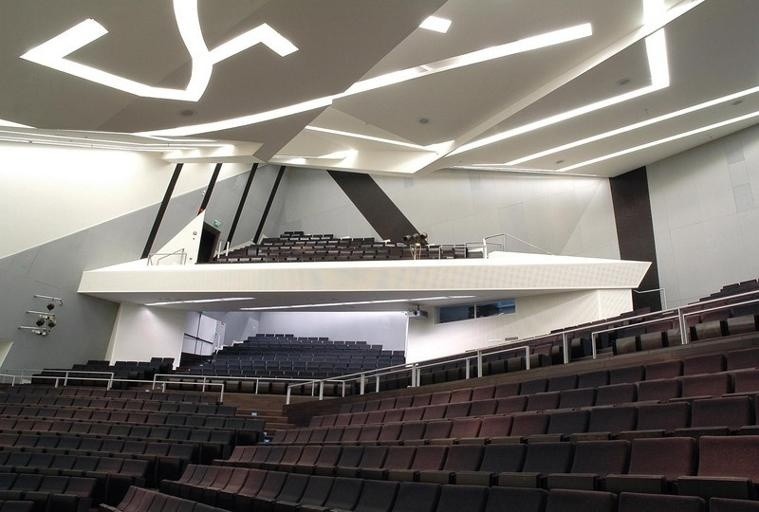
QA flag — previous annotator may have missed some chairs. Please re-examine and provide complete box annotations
[207,229,483,264]
[0,384,755,510]
[410,280,758,385]
[214,334,406,365]
[31,357,406,394]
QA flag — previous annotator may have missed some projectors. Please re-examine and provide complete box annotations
[405,309,428,319]
